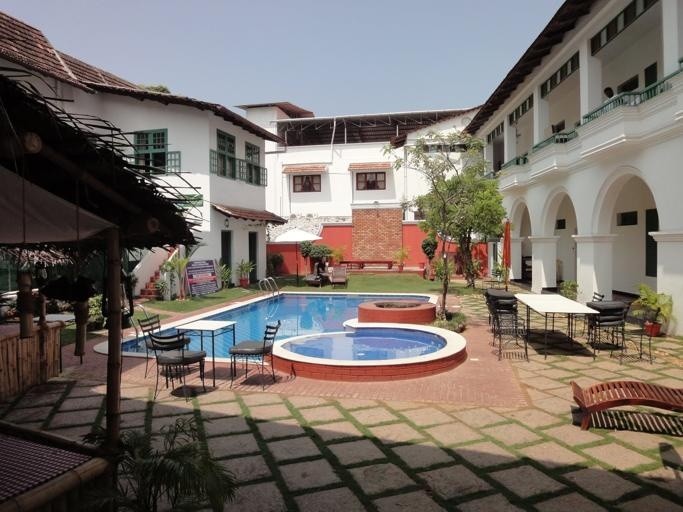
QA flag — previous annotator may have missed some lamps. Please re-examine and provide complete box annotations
[101,265,136,331]
[225,218,230,228]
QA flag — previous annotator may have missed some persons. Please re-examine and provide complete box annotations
[317,255,332,287]
[604,87,628,105]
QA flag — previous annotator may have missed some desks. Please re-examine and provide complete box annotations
[175,321,238,388]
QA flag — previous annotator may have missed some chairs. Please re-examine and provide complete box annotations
[228,320,282,391]
[147,331,207,402]
[482,287,661,366]
[137,314,192,378]
[331,266,349,289]
